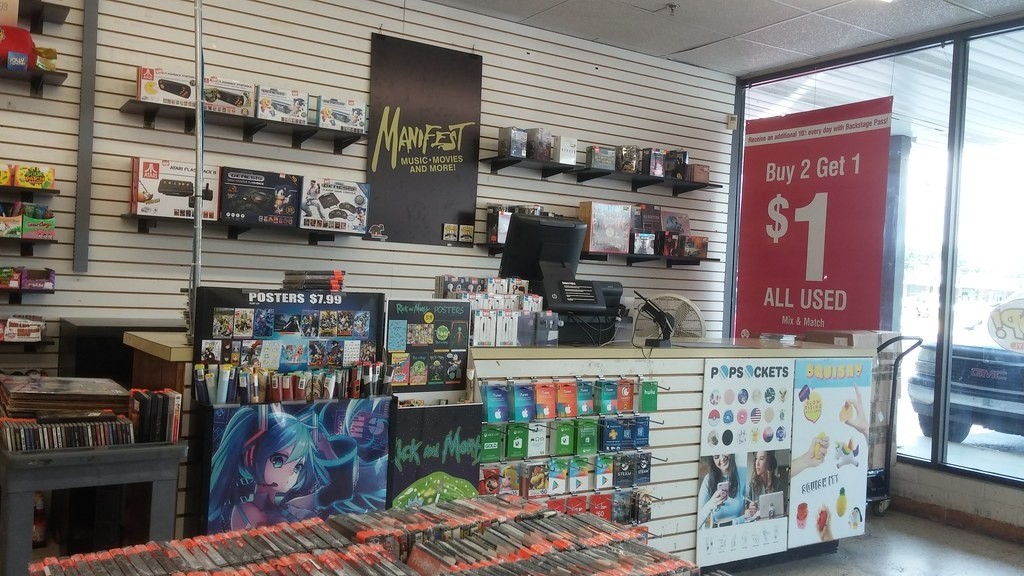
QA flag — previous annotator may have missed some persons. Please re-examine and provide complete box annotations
[697,453,748,528]
[745,450,788,520]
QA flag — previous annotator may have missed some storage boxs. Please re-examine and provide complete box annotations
[0,266,56,290]
[434,275,558,348]
[0,314,46,343]
[804,329,902,471]
[497,125,708,183]
[136,65,366,135]
[0,201,55,240]
[0,162,55,191]
[129,156,370,235]
[579,200,709,259]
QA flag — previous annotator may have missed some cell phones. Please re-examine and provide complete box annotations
[717,481,728,500]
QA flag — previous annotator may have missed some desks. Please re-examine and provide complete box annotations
[58,318,190,390]
[0,441,188,576]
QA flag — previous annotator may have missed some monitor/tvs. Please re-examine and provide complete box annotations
[498,213,587,296]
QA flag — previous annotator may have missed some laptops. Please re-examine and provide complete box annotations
[759,491,784,518]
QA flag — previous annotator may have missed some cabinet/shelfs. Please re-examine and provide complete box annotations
[0,185,60,353]
[119,100,372,246]
[0,0,70,98]
[479,156,723,269]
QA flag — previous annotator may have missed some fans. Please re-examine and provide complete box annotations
[633,294,707,341]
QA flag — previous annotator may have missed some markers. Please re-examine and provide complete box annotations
[189,359,394,403]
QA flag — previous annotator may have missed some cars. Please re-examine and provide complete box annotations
[908,290,1024,443]
